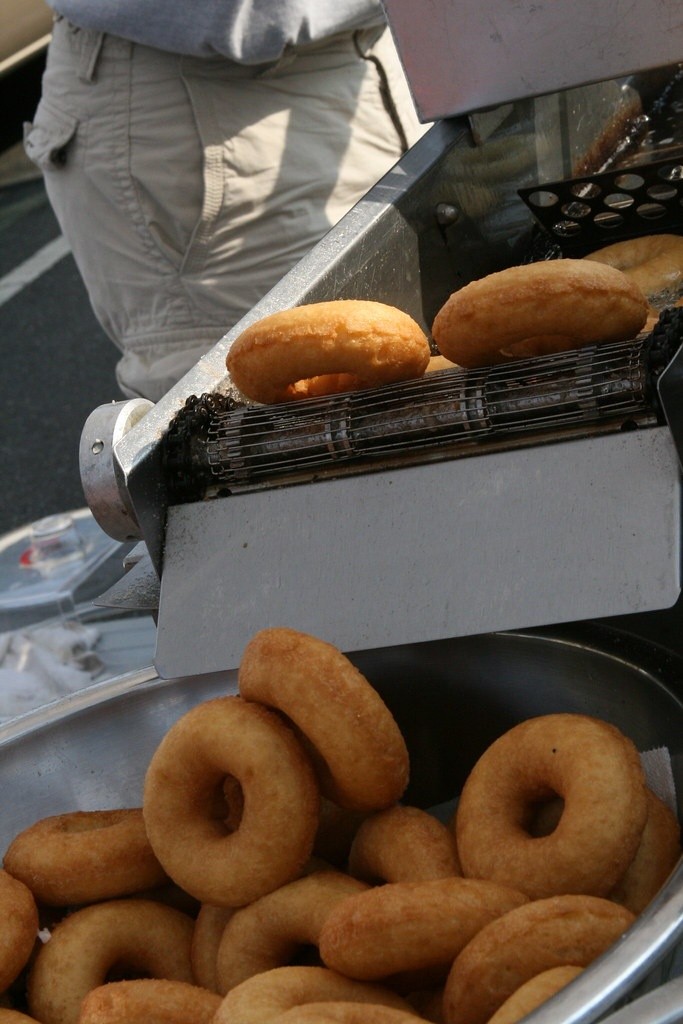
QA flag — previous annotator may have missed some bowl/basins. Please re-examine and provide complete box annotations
[0,629,682,1024]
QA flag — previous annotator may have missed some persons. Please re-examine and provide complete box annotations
[22,0,432,398]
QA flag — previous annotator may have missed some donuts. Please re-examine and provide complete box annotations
[224,233,683,401]
[0,625,680,1023]
